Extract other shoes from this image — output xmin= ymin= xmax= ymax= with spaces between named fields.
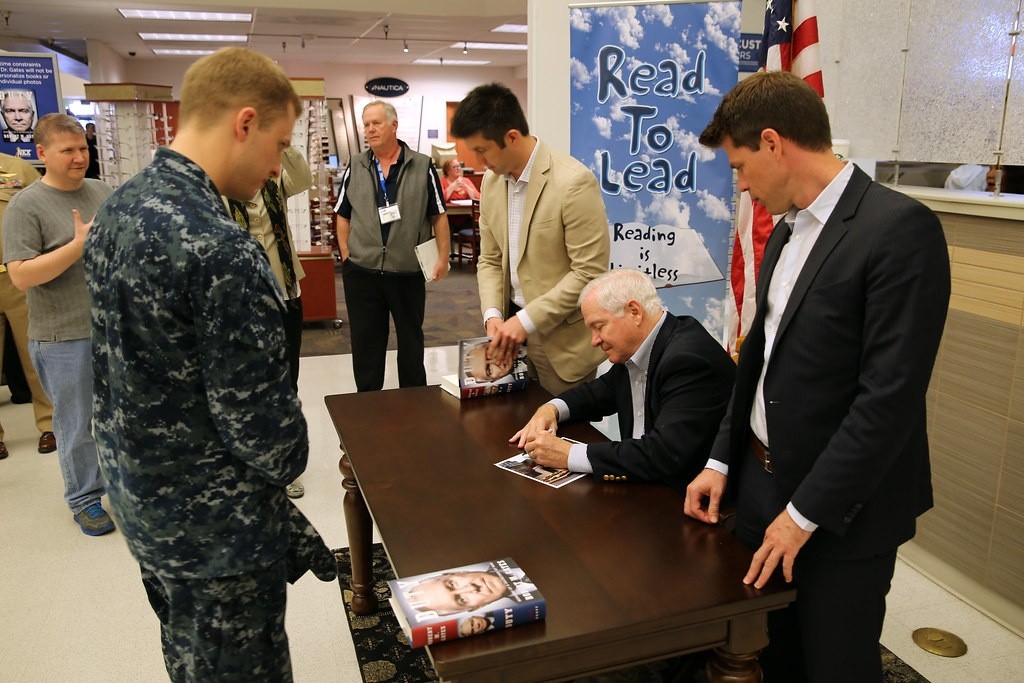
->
xmin=286 ymin=480 xmax=305 ymax=499
xmin=10 ymin=395 xmax=33 ymax=404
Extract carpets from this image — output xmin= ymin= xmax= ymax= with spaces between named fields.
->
xmin=330 ymin=538 xmax=934 ymax=683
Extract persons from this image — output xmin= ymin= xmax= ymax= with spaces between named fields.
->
xmin=684 ymin=71 xmax=950 ymax=683
xmin=408 ymin=559 xmax=542 ymax=616
xmin=220 ymin=139 xmax=313 ymax=499
xmin=450 ymin=79 xmax=611 ymax=396
xmin=333 ymin=98 xmax=451 ymax=393
xmin=459 ymin=616 xmax=490 ymax=637
xmin=83 ymin=46 xmax=310 ymax=683
xmin=85 ymin=123 xmax=101 ymax=180
xmin=0 ymin=153 xmax=57 ymax=460
xmin=2 ymin=113 xmax=115 ymax=536
xmin=985 ymin=165 xmax=1024 ymax=194
xmin=0 ymin=92 xmax=36 ymax=132
xmin=465 ymin=340 xmax=527 ymax=384
xmin=508 ymin=268 xmax=737 ymax=493
xmin=440 ymin=157 xmax=480 ymax=202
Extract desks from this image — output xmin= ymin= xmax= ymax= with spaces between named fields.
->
xmin=321 ymin=376 xmax=802 ymax=682
xmin=443 ymin=200 xmax=483 ymax=264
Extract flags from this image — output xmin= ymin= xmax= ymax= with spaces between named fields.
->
xmin=731 ymin=0 xmax=826 ymax=351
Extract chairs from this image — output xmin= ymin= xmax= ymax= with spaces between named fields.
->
xmin=450 ymin=200 xmax=480 ymax=272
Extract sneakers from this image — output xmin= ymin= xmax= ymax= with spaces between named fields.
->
xmin=73 ymin=503 xmax=116 ymax=536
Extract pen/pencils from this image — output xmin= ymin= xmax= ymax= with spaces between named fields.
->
xmin=522 ymin=429 xmax=553 ymax=456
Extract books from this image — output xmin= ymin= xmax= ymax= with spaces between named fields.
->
xmin=386 ymin=557 xmax=547 ymax=650
xmin=439 ymin=337 xmax=527 ymax=400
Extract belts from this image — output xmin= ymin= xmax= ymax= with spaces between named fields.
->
xmin=752 ymin=441 xmax=773 ymax=474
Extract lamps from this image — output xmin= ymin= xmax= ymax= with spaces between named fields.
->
xmin=402 ymin=37 xmax=409 ymax=53
xmin=463 ymin=41 xmax=468 ymax=55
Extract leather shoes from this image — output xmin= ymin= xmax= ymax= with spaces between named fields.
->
xmin=0 ymin=442 xmax=8 ymax=460
xmin=38 ymin=432 xmax=56 ymax=453
